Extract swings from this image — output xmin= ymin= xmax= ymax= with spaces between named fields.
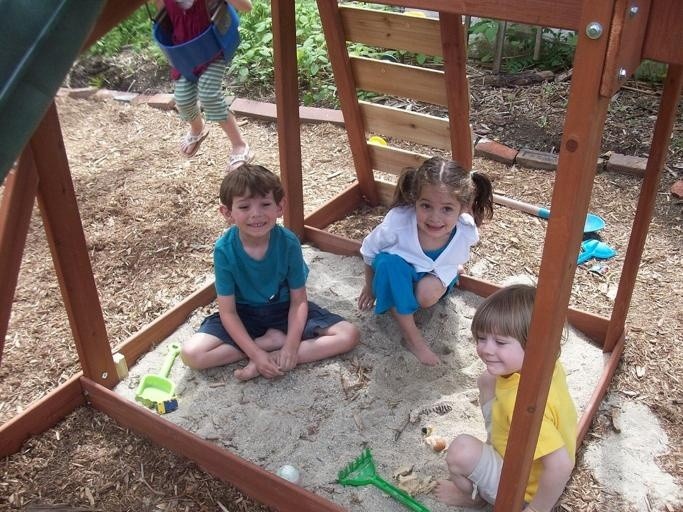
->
xmin=144 ymin=0 xmax=238 ymax=80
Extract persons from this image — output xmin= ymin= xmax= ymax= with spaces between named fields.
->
xmin=178 ymin=156 xmax=360 ymax=382
xmin=431 ymin=284 xmax=580 ymax=512
xmin=151 ymin=0 xmax=255 ymax=175
xmin=358 ymin=155 xmax=495 ymax=367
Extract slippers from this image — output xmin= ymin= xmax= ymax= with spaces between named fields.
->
xmin=181 ymin=119 xmax=210 ymax=158
xmin=226 ymin=142 xmax=255 ymax=173
